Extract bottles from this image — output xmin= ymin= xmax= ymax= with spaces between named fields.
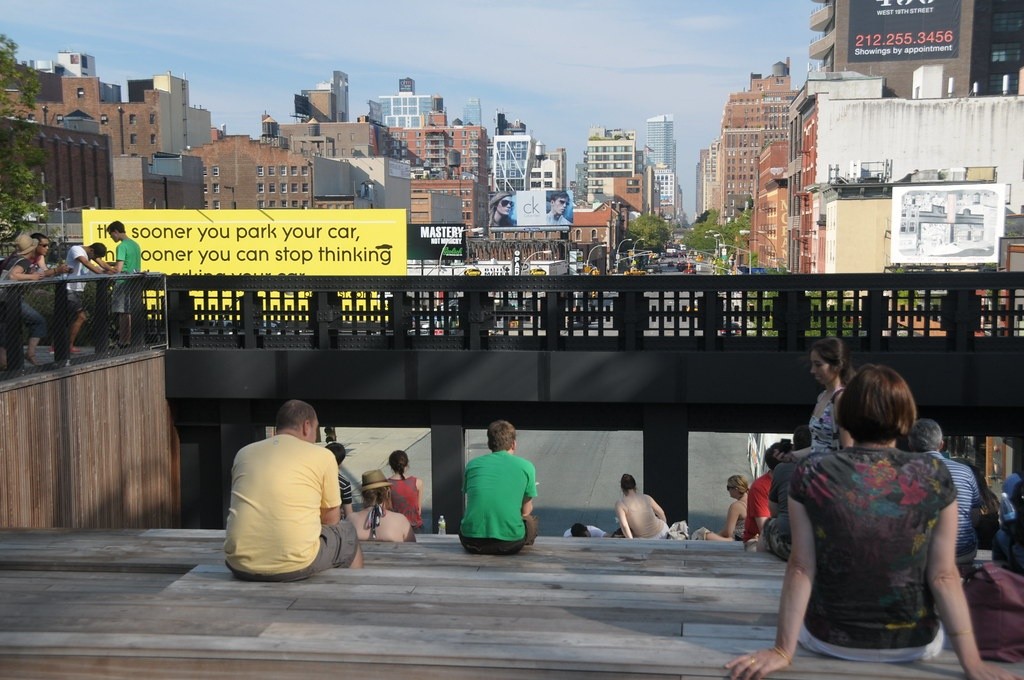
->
xmin=60 ymin=259 xmax=67 ymax=278
xmin=36 ymin=265 xmax=45 ymax=273
xmin=438 ymin=515 xmax=446 ymax=536
xmin=1001 ymin=493 xmax=1016 ymax=521
xmin=994 ymin=440 xmax=999 ymax=451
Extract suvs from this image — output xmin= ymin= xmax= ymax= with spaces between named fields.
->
xmin=647 ymin=265 xmax=661 ymax=273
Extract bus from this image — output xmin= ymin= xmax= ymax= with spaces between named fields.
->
xmin=680 ymin=245 xmax=686 ymax=251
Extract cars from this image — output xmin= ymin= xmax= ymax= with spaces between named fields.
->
xmin=721 ymin=323 xmax=740 ymax=336
xmin=624 ymin=267 xmax=646 ymax=275
xmin=668 ymin=262 xmax=674 ymax=266
xmin=572 ymin=315 xmax=592 ymax=329
xmin=683 ymin=262 xmax=696 ymax=274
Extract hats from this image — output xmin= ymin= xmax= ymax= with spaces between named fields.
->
xmin=15 ymin=233 xmax=39 ymax=254
xmin=358 ymin=469 xmax=391 ymax=490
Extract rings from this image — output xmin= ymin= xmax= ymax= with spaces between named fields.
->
xmin=750 ymin=657 xmax=756 ymax=665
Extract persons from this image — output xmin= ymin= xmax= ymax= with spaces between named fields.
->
xmin=725 ymin=364 xmax=1024 ymax=680
xmin=616 ymin=475 xmax=670 ymax=539
xmin=324 ymin=442 xmax=352 ymax=523
xmin=706 ymin=476 xmax=751 ymax=540
xmin=487 ymin=191 xmax=517 ymax=227
xmin=384 ymin=450 xmax=425 ymax=534
xmin=51 ymin=243 xmax=119 ymax=356
xmin=224 ymin=399 xmax=363 ymax=582
xmin=742 ymin=338 xmax=1023 ymax=576
xmin=562 ymin=522 xmax=610 ymax=538
xmin=460 ymin=421 xmax=538 ymax=555
xmin=546 ymin=190 xmax=573 ymax=225
xmin=345 ymin=470 xmax=416 ymax=543
xmin=0 ymin=233 xmax=70 ymax=371
xmin=106 ymin=221 xmax=141 ymax=350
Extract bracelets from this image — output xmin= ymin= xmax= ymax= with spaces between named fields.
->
xmin=53 ymin=269 xmax=57 ymax=273
xmin=773 ymin=647 xmax=792 ymax=665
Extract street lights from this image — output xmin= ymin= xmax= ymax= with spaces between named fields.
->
xmin=705 ymin=230 xmax=725 ymax=274
xmin=617 ymin=239 xmax=633 ymax=274
xmin=521 ymin=250 xmax=551 ymax=274
xmin=740 ymin=230 xmax=779 ymax=272
xmin=633 ymin=238 xmax=644 ymax=267
xmin=719 ymin=244 xmax=752 ymax=274
xmin=587 ymin=244 xmax=607 ymax=275
xmin=439 ymin=227 xmax=484 ymax=276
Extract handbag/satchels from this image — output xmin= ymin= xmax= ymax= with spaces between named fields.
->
xmin=0 ymin=258 xmax=29 ymax=302
xmin=957 ymin=560 xmax=1024 ymax=662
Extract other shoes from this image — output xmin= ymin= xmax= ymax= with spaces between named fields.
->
xmin=69 ymin=345 xmax=82 ymax=354
xmin=50 ymin=347 xmax=54 ymax=353
xmin=25 ymin=352 xmax=40 ymax=366
xmin=110 ymin=343 xmax=129 ymax=349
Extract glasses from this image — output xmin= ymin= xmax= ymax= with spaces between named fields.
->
xmin=557 ymin=199 xmax=571 ymax=206
xmin=501 ymin=199 xmax=515 ymax=209
xmin=726 ymin=486 xmax=736 ymax=490
xmin=38 ymin=243 xmax=50 ymax=248
